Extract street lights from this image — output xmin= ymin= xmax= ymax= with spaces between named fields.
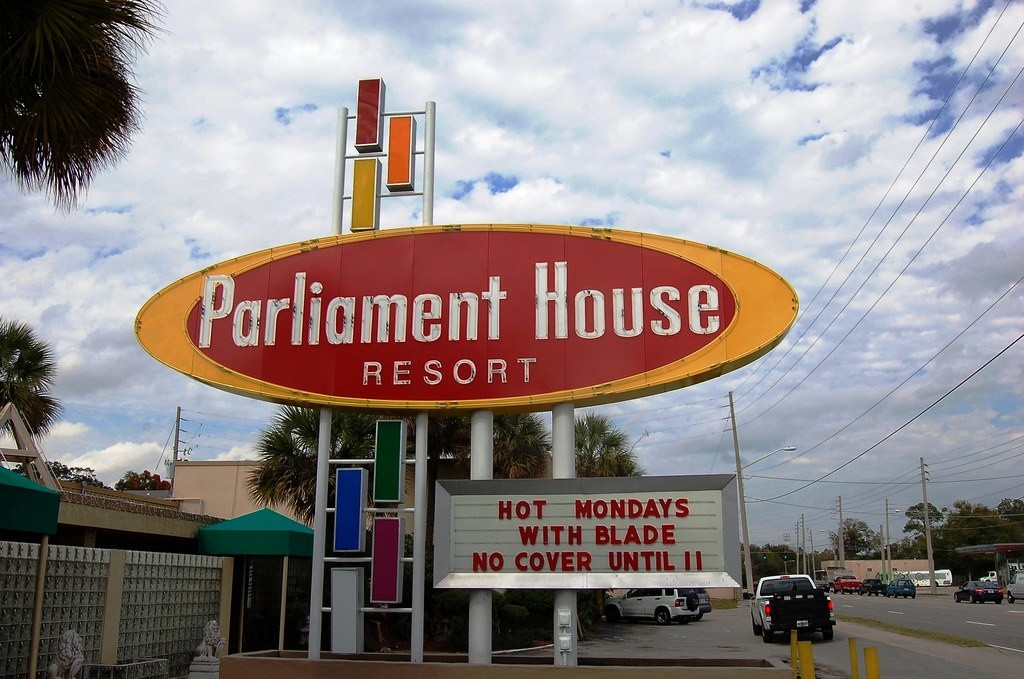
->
xmin=734 ymin=446 xmax=797 ymax=598
xmin=894 ymin=509 xmax=937 ymax=594
xmin=820 ymin=530 xmax=845 ymax=567
xmin=783 ymin=560 xmax=795 ymax=574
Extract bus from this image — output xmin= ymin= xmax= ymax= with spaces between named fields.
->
xmin=875 ymin=569 xmax=952 ymax=587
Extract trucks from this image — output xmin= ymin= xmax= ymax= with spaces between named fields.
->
xmin=1006 ymin=573 xmax=1024 ymax=604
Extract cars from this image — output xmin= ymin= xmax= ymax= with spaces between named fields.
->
xmin=886 ymin=579 xmax=916 ymax=598
xmin=815 ymin=580 xmax=830 ymax=593
xmin=954 ymin=581 xmax=1004 ymax=604
xmin=978 ymin=571 xmax=998 ymax=582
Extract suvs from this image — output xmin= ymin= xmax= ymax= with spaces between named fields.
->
xmin=603 ymin=588 xmax=712 ymax=626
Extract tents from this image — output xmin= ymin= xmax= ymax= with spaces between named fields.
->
xmin=197 ymin=508 xmax=313 ymax=653
xmin=0 ymin=465 xmax=61 ymax=679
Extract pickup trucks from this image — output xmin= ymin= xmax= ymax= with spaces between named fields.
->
xmin=858 ymin=579 xmax=888 ymax=596
xmin=833 ymin=575 xmax=863 ymax=594
xmin=750 ymin=574 xmax=836 ymax=643
